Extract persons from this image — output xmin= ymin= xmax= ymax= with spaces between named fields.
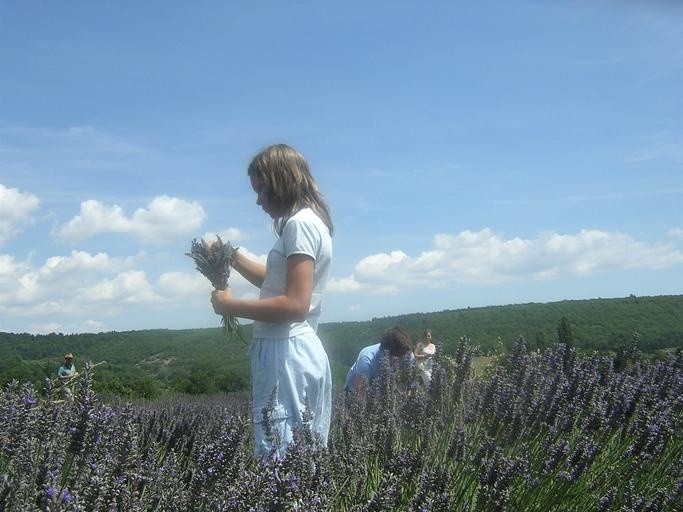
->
xmin=344 ymin=326 xmax=417 ymax=399
xmin=412 ymin=328 xmax=437 ymax=389
xmin=199 ymin=143 xmax=335 ymax=469
xmin=56 ymin=352 xmax=79 ymax=403
